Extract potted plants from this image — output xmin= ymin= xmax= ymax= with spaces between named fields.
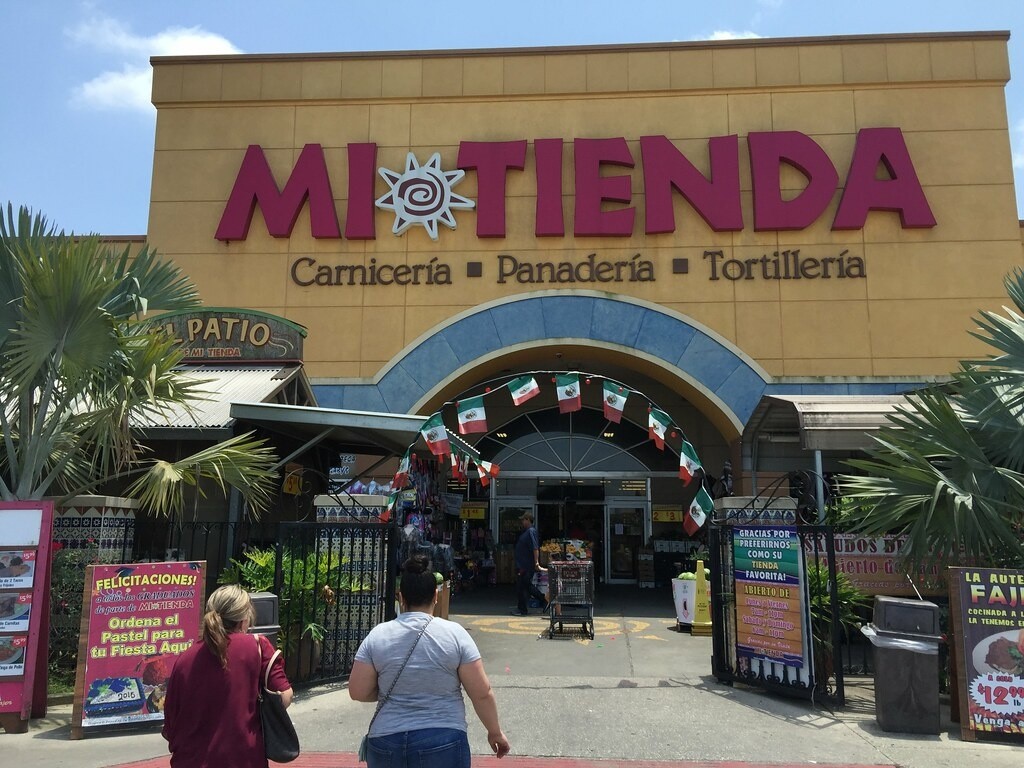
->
xmin=214 ymin=543 xmax=375 ymax=682
xmin=806 ymin=556 xmax=875 ymax=693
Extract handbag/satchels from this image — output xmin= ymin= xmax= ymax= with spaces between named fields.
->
xmin=514 ymin=568 xmax=526 ymax=577
xmin=252 ymin=632 xmax=299 ymax=764
xmin=356 ymin=735 xmax=367 ymax=761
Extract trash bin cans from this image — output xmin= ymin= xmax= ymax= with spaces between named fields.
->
xmin=248 ymin=591 xmax=283 ymax=651
xmin=869 ymin=593 xmax=945 ymax=736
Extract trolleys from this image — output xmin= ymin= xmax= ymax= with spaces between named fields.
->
xmin=546 ymin=560 xmax=596 ymax=639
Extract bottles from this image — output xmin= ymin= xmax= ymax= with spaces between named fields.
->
xmin=366 ymin=478 xmax=378 ymax=495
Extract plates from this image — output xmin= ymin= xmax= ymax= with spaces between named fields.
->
xmin=0 ymin=647 xmax=23 ymax=663
xmin=972 ymin=630 xmax=1021 ymax=675
xmin=0 ymin=594 xmax=28 ymax=620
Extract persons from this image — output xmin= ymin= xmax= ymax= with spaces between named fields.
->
xmin=590 ymin=522 xmax=605 ymax=582
xmin=538 ymin=553 xmax=563 ymax=616
xmin=163 ymin=584 xmax=293 ymax=768
xmin=512 ymin=511 xmax=550 ymax=617
xmin=559 ymin=519 xmax=588 ymax=540
xmin=349 ymin=554 xmax=510 ymax=768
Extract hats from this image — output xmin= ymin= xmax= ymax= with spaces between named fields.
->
xmin=518 ymin=512 xmax=535 ymax=520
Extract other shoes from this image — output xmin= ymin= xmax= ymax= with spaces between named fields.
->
xmin=543 ymin=601 xmax=550 ymax=613
xmin=512 ymin=611 xmax=528 ymax=617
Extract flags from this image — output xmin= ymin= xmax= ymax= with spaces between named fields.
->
xmin=648 ymin=404 xmax=673 ymax=451
xmin=439 ymin=444 xmax=500 ymax=487
xmin=603 ymin=380 xmax=630 ymax=424
xmin=683 ymin=488 xmax=713 ymax=537
xmin=679 ymin=440 xmax=706 ymax=487
xmin=420 ymin=413 xmax=451 ymax=455
xmin=377 ymin=447 xmax=410 ymax=525
xmin=555 ymin=373 xmax=582 ymax=414
xmin=507 ymin=373 xmax=540 ymax=407
xmin=455 ymin=397 xmax=488 ymax=435
